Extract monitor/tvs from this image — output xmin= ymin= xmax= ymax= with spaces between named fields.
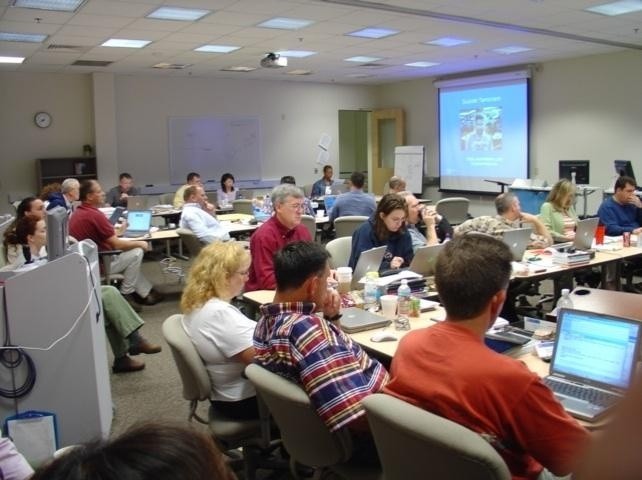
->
xmin=47 ymin=206 xmax=69 ymax=262
xmin=559 ymin=160 xmax=589 ymax=186
xmin=614 ymin=160 xmax=636 ymax=185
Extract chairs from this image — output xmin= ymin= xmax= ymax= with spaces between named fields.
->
xmin=0 ymin=179 xmax=642 ymax=322
xmin=243 ymin=355 xmax=361 ymax=480
xmin=360 ymin=391 xmax=515 ymax=480
xmin=159 ymin=307 xmax=316 ymax=480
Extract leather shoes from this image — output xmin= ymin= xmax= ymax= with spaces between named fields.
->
xmin=113 ymin=359 xmax=145 ymax=372
xmin=129 ymin=340 xmax=161 ymax=355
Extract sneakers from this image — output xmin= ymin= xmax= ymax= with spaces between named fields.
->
xmin=123 ymin=293 xmax=142 ymax=313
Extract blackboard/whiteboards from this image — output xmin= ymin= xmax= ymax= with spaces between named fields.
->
xmin=168 ymin=116 xmax=265 ymax=189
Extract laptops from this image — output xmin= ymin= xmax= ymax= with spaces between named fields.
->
xmin=327 ymin=245 xmax=388 ymax=291
xmin=314 ymin=307 xmax=392 ymax=334
xmin=549 ymin=216 xmax=600 ymax=250
xmin=324 ymin=195 xmax=338 ymax=215
xmin=502 ymin=228 xmax=533 ymax=261
xmin=127 ymin=197 xmax=148 ymax=210
xmin=540 ymin=308 xmax=642 ymax=422
xmin=123 ymin=210 xmax=152 ymax=237
xmin=332 ymin=185 xmax=348 ymax=195
xmin=109 ymin=208 xmax=126 ymax=226
xmin=397 ymin=243 xmax=445 ymax=276
xmin=235 ymin=191 xmax=253 ymax=200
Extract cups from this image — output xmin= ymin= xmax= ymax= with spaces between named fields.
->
xmin=336 ymin=266 xmax=353 ymax=294
xmin=595 ymin=224 xmax=606 ymax=245
xmin=317 ymin=209 xmax=325 ymax=219
xmin=380 ymin=293 xmax=398 ymax=317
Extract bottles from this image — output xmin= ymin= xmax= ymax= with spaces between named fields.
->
xmin=263 ymin=194 xmax=273 ymax=219
xmin=397 ymin=279 xmax=412 ymax=319
xmin=251 ymin=196 xmax=265 ymax=220
xmin=554 ymin=286 xmax=574 ymax=319
xmin=362 ymin=272 xmax=379 ymax=307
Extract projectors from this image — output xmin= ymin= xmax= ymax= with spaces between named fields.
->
xmin=261 ymin=56 xmax=287 ymax=68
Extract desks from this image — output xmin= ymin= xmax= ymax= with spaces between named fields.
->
xmin=235 ymin=284 xmax=641 ymax=437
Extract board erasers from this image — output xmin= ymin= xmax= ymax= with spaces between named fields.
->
xmin=145 ymin=184 xmax=153 ymax=188
xmin=207 ymin=179 xmax=214 ymax=183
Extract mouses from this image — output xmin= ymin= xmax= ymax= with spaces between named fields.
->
xmin=371 ymin=333 xmax=397 ymax=342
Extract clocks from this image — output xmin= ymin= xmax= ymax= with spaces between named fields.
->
xmin=33 ymin=111 xmax=53 ymax=129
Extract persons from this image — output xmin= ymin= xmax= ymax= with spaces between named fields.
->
xmin=459 ymin=112 xmax=503 ymax=151
xmin=29 ymin=416 xmax=239 ymax=480
xmin=180 ymin=238 xmax=265 ymax=422
xmin=382 ymin=232 xmax=590 ymax=480
xmin=5 ymin=164 xmax=641 ymax=377
xmin=252 ymin=239 xmax=390 ymax=480
